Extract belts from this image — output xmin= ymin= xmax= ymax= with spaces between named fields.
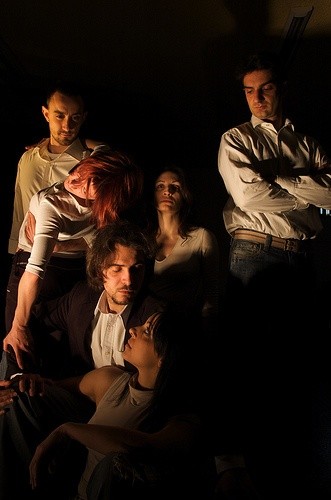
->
xmin=234 ymin=229 xmax=298 ymax=251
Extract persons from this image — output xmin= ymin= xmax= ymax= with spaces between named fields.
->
xmin=0 ymin=57 xmax=331 ymax=500
